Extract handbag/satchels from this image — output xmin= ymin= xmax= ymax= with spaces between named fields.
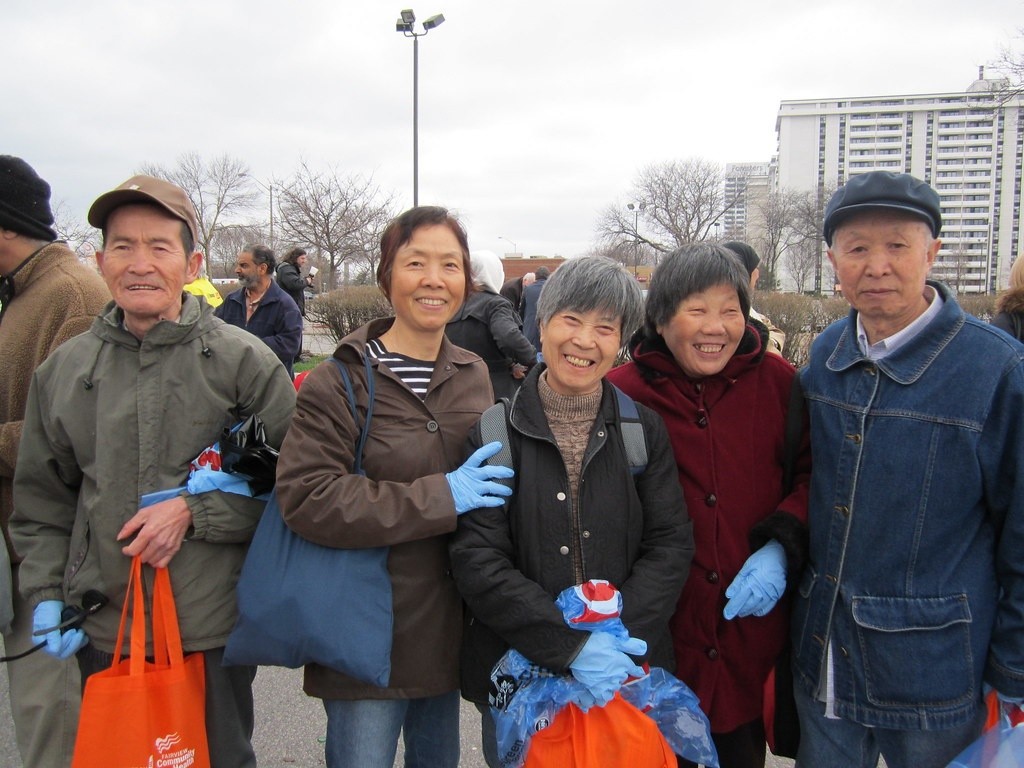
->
xmin=764 ymin=645 xmax=800 ymax=760
xmin=70 ymin=554 xmax=212 ymax=768
xmin=221 ymin=356 xmax=393 ymax=692
xmin=944 ymin=690 xmax=1024 ymax=768
xmin=519 ymin=663 xmax=680 ymax=768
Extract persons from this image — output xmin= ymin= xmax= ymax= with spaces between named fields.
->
xmin=213 ymin=242 xmax=312 ymax=381
xmin=796 ymin=172 xmax=1024 ymax=768
xmin=604 ymin=240 xmax=810 ymax=768
xmin=7 ymin=175 xmax=314 ymax=768
xmin=0 ymin=156 xmax=114 ymax=768
xmin=276 ymin=206 xmax=692 ymax=768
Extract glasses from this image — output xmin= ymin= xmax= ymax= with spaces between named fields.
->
xmin=0 ymin=588 xmax=110 ymax=663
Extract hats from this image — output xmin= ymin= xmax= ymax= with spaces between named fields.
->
xmin=823 ymin=170 xmax=942 ymax=248
xmin=88 ymin=175 xmax=200 ymax=249
xmin=1 ymin=155 xmax=58 ymax=242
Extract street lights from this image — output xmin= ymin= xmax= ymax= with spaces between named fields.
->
xmin=396 ymin=9 xmax=445 ymax=208
xmin=498 ymin=236 xmax=516 ymax=254
xmin=714 ymin=223 xmax=720 ymax=241
xmin=627 ymin=203 xmax=646 ymax=279
xmin=238 ymin=173 xmax=274 ymax=251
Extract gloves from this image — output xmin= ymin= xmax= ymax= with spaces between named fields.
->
xmin=570 ymin=631 xmax=648 ymax=703
xmin=443 ymin=441 xmax=516 ymax=515
xmin=724 ymin=540 xmax=788 ymax=618
xmin=31 ymin=600 xmax=90 ymax=659
